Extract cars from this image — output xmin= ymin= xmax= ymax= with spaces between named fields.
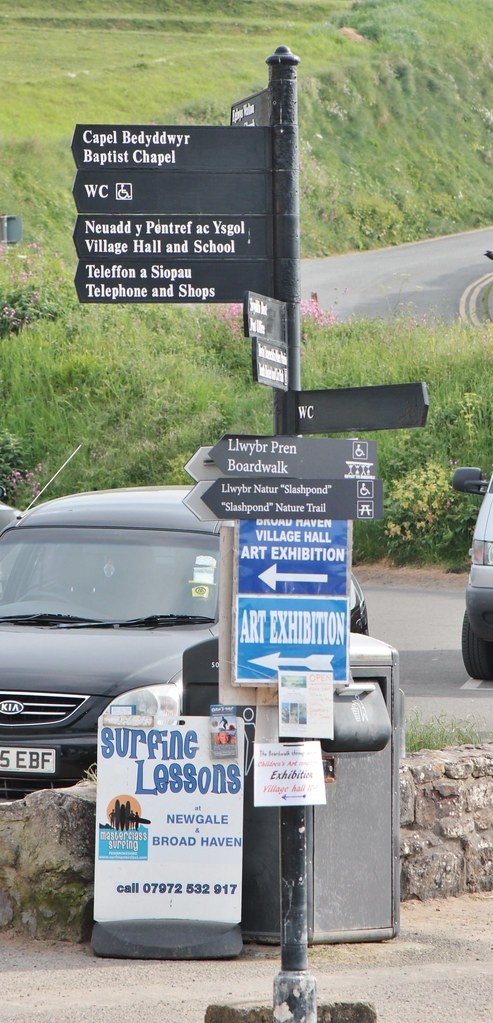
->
xmin=0 ymin=485 xmax=370 ymax=802
xmin=451 ymin=466 xmax=493 ymax=682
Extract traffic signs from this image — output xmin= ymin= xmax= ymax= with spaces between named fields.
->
xmin=180 ymin=432 xmax=379 ymax=478
xmin=179 ymin=478 xmax=384 ymax=521
xmin=69 ymin=124 xmax=277 ymax=302
xmin=230 ymin=594 xmax=352 ymax=684
xmin=236 ymin=520 xmax=354 ymax=598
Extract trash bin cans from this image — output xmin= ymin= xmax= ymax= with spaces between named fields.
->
xmin=241 ymin=632 xmax=405 ymax=948
